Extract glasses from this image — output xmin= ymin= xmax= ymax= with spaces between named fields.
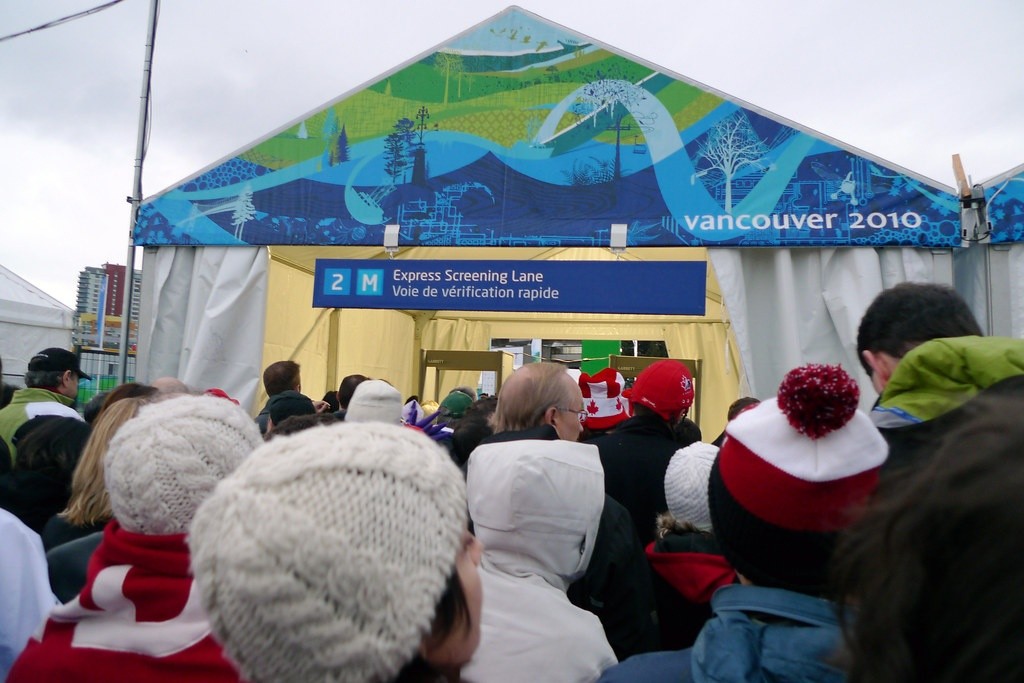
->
xmin=541 ymin=407 xmax=589 ymax=422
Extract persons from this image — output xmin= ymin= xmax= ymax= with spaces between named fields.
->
xmin=0 ymin=283 xmax=1024 ymax=683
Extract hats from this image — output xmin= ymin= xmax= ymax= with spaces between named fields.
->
xmin=27 ymin=347 xmax=93 ymax=382
xmin=203 ymin=388 xmax=240 ymax=406
xmin=707 ymin=363 xmax=889 ymax=593
xmin=439 ymin=392 xmax=475 ymax=419
xmin=267 ymin=391 xmax=317 ymax=427
xmin=577 ymin=367 xmax=631 ymax=427
xmin=344 ymin=379 xmax=402 ymax=425
xmin=186 ymin=419 xmax=465 ymax=683
xmin=104 ymin=393 xmax=264 ymax=536
xmin=663 ymin=442 xmax=719 ymax=533
xmin=622 ymin=358 xmax=694 ymax=427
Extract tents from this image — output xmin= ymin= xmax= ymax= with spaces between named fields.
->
xmin=0 ymin=6 xmax=1024 ymax=404
